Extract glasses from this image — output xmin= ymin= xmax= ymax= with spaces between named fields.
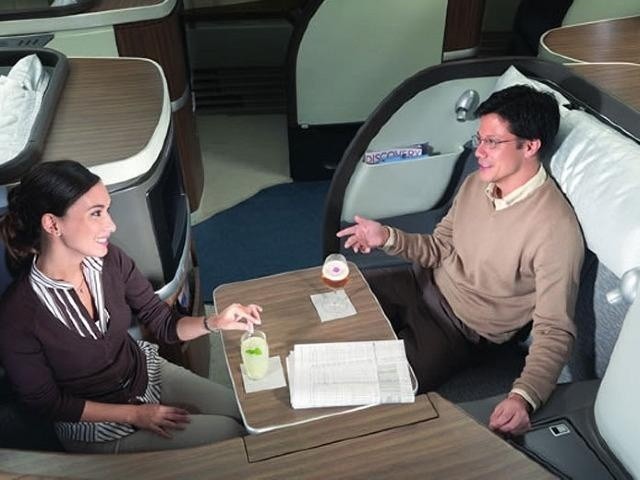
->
xmin=471 ymin=134 xmax=520 ymax=149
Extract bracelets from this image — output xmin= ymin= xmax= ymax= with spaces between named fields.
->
xmin=203 ymin=317 xmax=215 ymax=333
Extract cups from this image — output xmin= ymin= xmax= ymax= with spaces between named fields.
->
xmin=241 ymin=330 xmax=272 ymax=379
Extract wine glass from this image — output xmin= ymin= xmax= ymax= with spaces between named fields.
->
xmin=320 ymin=254 xmax=351 ymax=311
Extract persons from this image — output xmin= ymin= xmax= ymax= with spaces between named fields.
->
xmin=1 ymin=159 xmax=264 ymax=455
xmin=336 ymin=83 xmax=585 ymax=437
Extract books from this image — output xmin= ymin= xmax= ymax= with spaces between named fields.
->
xmin=365 ymin=144 xmax=422 ymax=163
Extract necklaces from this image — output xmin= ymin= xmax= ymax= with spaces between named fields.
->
xmin=75 ymin=278 xmax=86 ymax=294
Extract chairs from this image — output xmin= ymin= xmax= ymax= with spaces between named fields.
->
xmin=324 ymin=57 xmax=639 ymax=404
